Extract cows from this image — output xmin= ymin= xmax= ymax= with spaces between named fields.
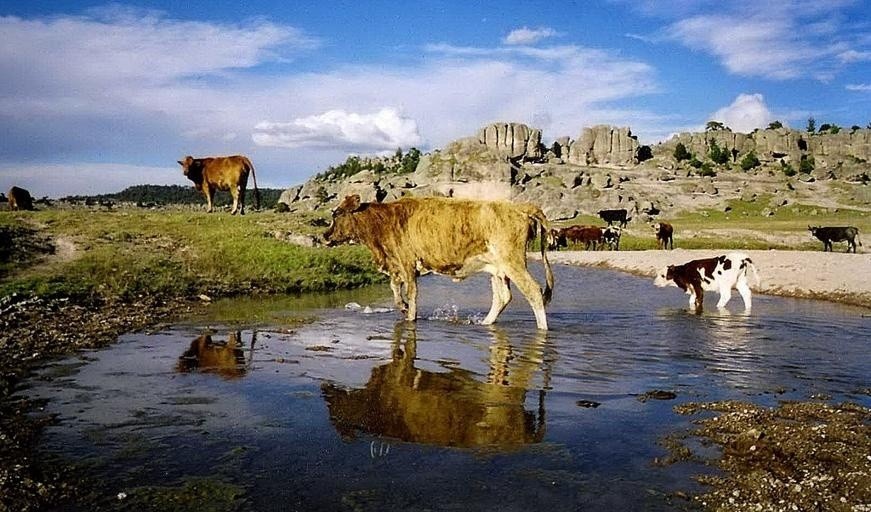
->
xmin=597 ymin=209 xmax=632 ymax=228
xmin=169 ymin=325 xmax=257 ymax=382
xmin=545 ymin=225 xmax=622 ymax=251
xmin=177 ymin=156 xmax=261 ymax=215
xmin=319 ymin=317 xmax=552 ymax=454
xmin=807 ymin=224 xmax=862 ymax=253
xmin=8 ymin=185 xmax=33 ymax=212
xmin=320 ymin=193 xmax=555 ymax=332
xmin=653 ymin=253 xmax=761 ymax=311
xmin=651 ymin=222 xmax=673 ymax=250
